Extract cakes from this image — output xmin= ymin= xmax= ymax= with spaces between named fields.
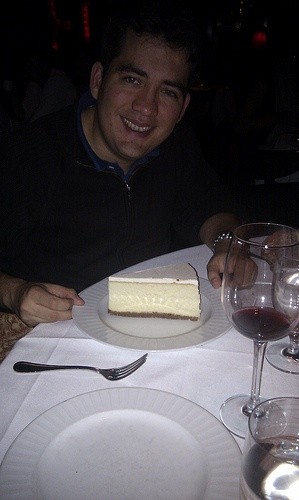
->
xmin=107 ymin=263 xmax=201 ymax=322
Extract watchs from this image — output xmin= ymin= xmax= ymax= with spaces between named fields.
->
xmin=212 ymin=231 xmax=250 ymax=253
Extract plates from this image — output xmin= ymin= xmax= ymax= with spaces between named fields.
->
xmin=72 ymin=277 xmax=234 ymax=352
xmin=0 ymin=386 xmax=245 ymax=500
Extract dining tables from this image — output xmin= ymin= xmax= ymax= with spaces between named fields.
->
xmin=0 ymin=234 xmax=299 ymax=500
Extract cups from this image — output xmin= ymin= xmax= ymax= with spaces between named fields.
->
xmin=240 ymin=397 xmax=299 ymax=500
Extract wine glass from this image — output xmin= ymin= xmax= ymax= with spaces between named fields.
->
xmin=220 ymin=223 xmax=299 ymax=439
xmin=265 ymin=252 xmax=299 ymax=374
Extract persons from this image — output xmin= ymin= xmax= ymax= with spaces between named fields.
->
xmin=0 ymin=12 xmax=258 ymax=327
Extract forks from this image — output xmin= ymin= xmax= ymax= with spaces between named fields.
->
xmin=13 ymin=353 xmax=148 ymax=381
